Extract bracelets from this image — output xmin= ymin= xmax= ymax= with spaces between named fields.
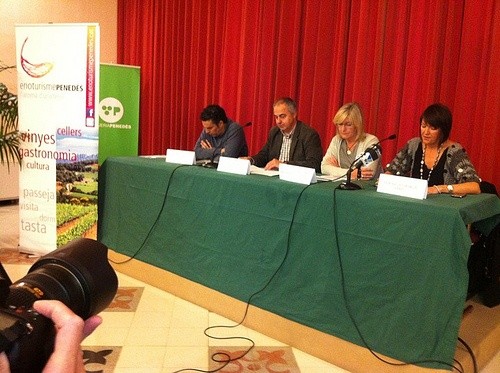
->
xmin=434 ymin=184 xmax=442 ymax=193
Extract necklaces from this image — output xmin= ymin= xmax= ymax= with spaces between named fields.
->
xmin=420 ymin=142 xmax=443 ymax=181
xmin=346 ymin=140 xmax=358 ymax=155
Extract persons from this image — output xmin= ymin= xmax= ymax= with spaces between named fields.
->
xmin=383 ymin=103 xmax=481 ymax=195
xmin=0 ymin=300 xmax=103 ymax=373
xmin=321 ymin=103 xmax=382 ymax=181
xmin=194 ymin=104 xmax=248 ymax=160
xmin=239 ymin=97 xmax=323 ymax=174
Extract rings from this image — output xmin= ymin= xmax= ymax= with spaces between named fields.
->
xmin=362 ymin=175 xmax=364 ymax=178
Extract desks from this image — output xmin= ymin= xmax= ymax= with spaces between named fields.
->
xmin=96 ymin=154 xmax=500 ymax=370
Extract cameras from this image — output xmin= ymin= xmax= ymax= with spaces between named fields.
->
xmin=0 ymin=236 xmax=119 ymax=373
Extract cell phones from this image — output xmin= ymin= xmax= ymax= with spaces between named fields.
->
xmin=451 ymin=193 xmax=468 ymax=198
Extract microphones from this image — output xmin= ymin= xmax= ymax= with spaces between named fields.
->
xmin=350 ymin=144 xmax=383 ymax=172
xmin=336 ymin=134 xmax=396 ymax=190
xmin=202 ymin=121 xmax=253 ymax=168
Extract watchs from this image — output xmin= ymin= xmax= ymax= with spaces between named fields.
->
xmin=447 ymin=183 xmax=453 ymax=193
